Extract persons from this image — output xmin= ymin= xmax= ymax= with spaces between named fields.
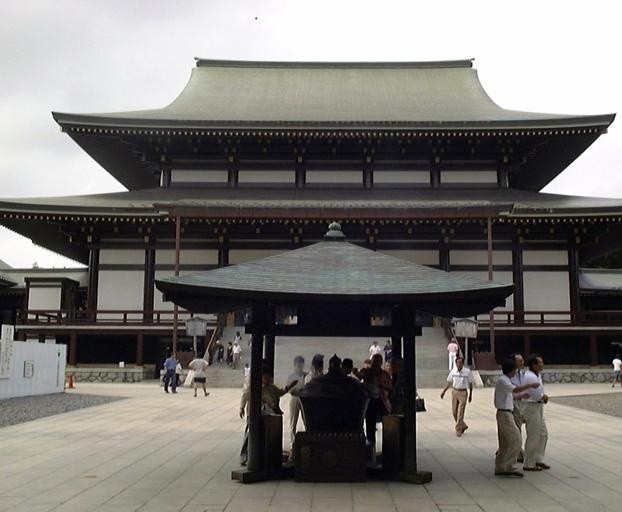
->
xmin=164 ymin=330 xmax=393 ymax=467
xmin=493 ymin=358 xmax=541 ymax=477
xmin=519 ymin=355 xmax=550 ymax=471
xmin=446 ymin=337 xmax=459 ymax=370
xmin=612 ymin=354 xmax=622 ymax=388
xmin=440 ymin=356 xmax=474 ymax=437
xmin=494 ymin=354 xmax=526 ymax=462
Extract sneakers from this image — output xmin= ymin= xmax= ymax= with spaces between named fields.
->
xmin=495 ymin=471 xmax=524 ymax=479
xmin=457 ymin=426 xmax=469 ymax=437
xmin=523 ymin=462 xmax=550 ymax=471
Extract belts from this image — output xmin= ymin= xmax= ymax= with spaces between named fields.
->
xmin=497 ymin=408 xmax=514 ymax=415
xmin=452 ymin=387 xmax=467 ymax=392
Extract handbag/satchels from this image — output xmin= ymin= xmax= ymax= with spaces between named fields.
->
xmin=415 ymin=394 xmax=426 ymax=413
